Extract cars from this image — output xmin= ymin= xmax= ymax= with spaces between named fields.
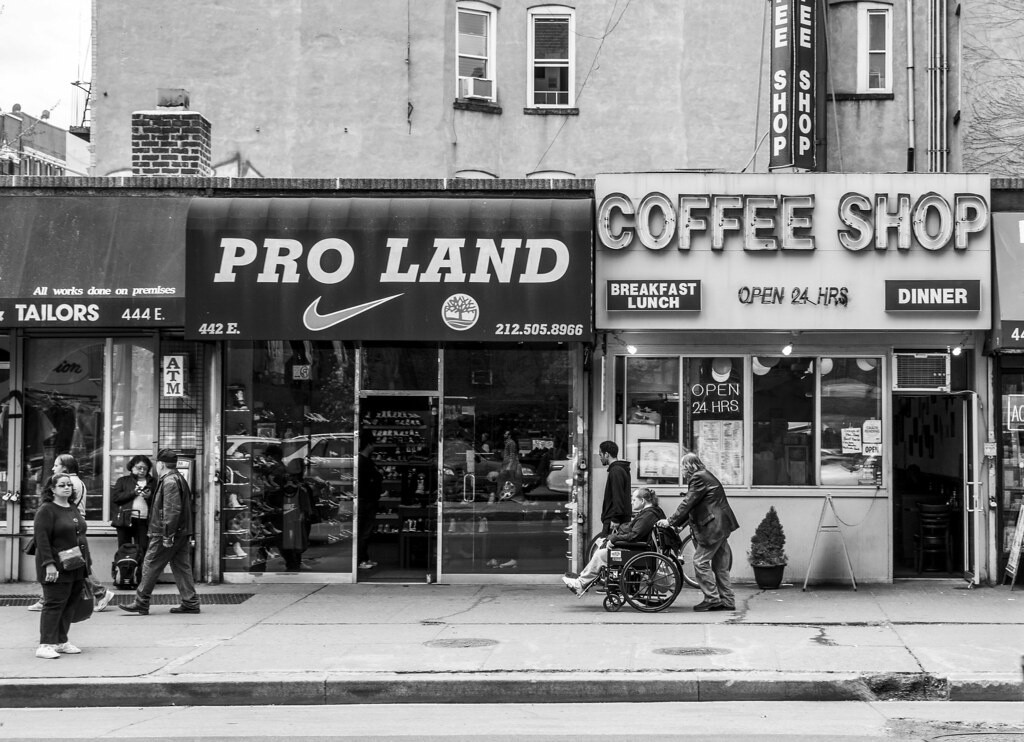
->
xmin=29 ymin=432 xmax=353 ymax=546
xmin=442 ymin=441 xmax=540 ymax=502
xmin=545 ymin=443 xmax=688 ymax=493
xmin=443 ymin=461 xmax=465 ymax=495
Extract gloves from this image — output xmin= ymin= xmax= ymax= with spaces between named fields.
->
xmin=267 ymin=340 xmax=285 ymax=374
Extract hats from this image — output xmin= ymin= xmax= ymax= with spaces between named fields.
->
xmin=151 ymin=448 xmax=177 ymax=464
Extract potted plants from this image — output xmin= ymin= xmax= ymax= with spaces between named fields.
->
xmin=747 ymin=505 xmax=788 ymax=589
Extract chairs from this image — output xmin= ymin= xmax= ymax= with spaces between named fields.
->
xmin=911 ymin=501 xmax=953 ymax=573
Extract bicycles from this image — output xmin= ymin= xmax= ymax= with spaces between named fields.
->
xmin=586 ymin=492 xmax=733 ymax=589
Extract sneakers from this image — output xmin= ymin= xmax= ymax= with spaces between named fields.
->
xmin=55 ymin=641 xmax=81 ymax=654
xmin=119 ymin=600 xmax=150 ymax=614
xmin=94 ymin=590 xmax=114 ymax=612
xmin=36 ymin=644 xmax=60 ymax=658
xmin=27 ymin=602 xmax=44 ymax=610
xmin=562 ymin=577 xmax=584 ymax=597
xmin=170 ymin=603 xmax=200 ymax=614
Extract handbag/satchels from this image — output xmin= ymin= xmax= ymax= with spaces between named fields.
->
xmin=57 ymin=545 xmax=86 ymax=570
xmin=111 ymin=510 xmax=133 ymax=529
xmin=70 ymin=581 xmax=93 ymax=623
xmin=23 ymin=538 xmax=37 ymax=556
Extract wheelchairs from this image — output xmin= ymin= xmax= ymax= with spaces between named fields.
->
xmin=599 ymin=522 xmax=685 ymax=613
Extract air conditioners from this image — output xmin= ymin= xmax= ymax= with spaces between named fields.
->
xmin=462 ymin=77 xmax=493 ymax=100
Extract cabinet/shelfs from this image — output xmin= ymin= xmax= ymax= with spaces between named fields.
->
xmin=304 ymin=457 xmax=438 ymax=537
xmin=221 ymin=408 xmax=279 ymax=569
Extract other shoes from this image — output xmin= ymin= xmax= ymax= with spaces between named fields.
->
xmin=708 ymin=603 xmax=735 ymax=611
xmin=595 ymin=588 xmax=609 ymax=594
xmin=223 ymin=385 xmax=518 ymax=570
xmin=693 ymin=599 xmax=723 ymax=611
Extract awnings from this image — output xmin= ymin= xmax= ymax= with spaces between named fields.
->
xmin=0 ymin=195 xmax=593 ymax=342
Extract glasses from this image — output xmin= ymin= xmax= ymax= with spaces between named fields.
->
xmin=54 ymin=483 xmax=74 ymax=489
xmin=134 ymin=465 xmax=149 ymax=472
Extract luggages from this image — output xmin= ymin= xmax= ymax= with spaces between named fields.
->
xmin=111 ymin=508 xmax=144 ymax=590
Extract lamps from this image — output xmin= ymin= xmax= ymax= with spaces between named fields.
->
xmin=613 ymin=333 xmax=637 ymax=354
xmin=952 ymin=337 xmax=969 ymax=355
xmin=711 ymin=357 xmax=731 ymax=382
xmin=752 ymin=356 xmax=781 ymax=375
xmin=782 ymin=341 xmax=794 ymax=355
xmin=855 ymin=358 xmax=878 ymax=371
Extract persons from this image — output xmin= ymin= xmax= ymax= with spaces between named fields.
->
xmin=492 ymin=431 xmax=525 ymax=504
xmin=358 ymin=430 xmax=382 ymax=569
xmin=655 ymin=453 xmax=740 ymax=611
xmin=111 ymin=454 xmax=157 ymax=554
xmin=28 ymin=454 xmax=114 ymax=611
xmin=33 ymin=473 xmax=92 ymax=658
xmin=119 ymin=448 xmax=201 ymax=615
xmin=561 ymin=488 xmax=666 ymax=593
xmin=598 ymin=441 xmax=631 ymax=593
